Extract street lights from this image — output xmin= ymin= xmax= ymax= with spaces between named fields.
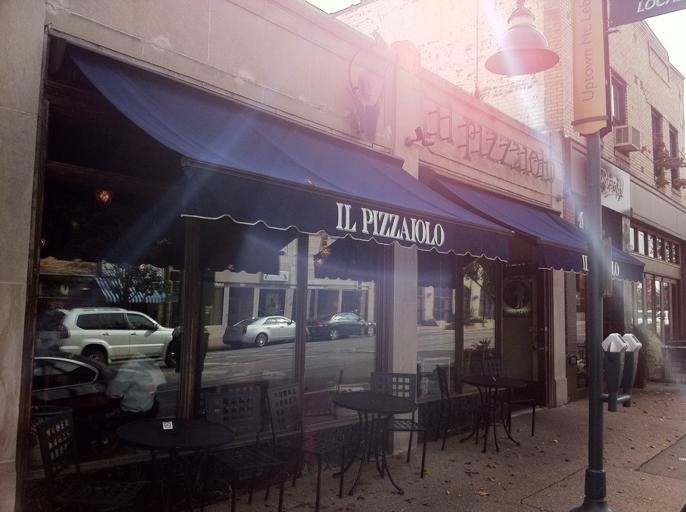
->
xmin=485 ymin=0 xmax=615 ymax=509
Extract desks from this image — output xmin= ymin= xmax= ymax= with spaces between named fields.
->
xmin=114 ymin=419 xmax=232 ymax=512
xmin=331 ymin=391 xmax=416 ymax=496
xmin=462 ymin=376 xmax=526 ymax=452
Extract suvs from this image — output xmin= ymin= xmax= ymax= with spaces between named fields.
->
xmin=37 ymin=304 xmax=181 ymax=369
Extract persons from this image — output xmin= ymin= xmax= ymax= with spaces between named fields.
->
xmin=85 ymin=334 xmax=168 ymax=447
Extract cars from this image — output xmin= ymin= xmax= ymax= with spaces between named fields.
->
xmin=30 ymin=354 xmax=155 ymax=413
xmin=310 ymin=310 xmax=379 ymax=340
xmin=227 ymin=315 xmax=310 ymax=345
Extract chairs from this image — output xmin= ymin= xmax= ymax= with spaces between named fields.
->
xmin=495 ymin=366 xmax=537 ymax=436
xmin=202 ymin=381 xmax=284 ymax=512
xmin=436 ymin=365 xmax=489 ymax=453
xmin=369 ymin=373 xmax=427 ymax=478
xmin=265 ymin=383 xmax=342 ymax=512
xmin=38 ymin=416 xmax=152 ymax=512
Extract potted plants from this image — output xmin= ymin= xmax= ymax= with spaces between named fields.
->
xmin=627 ymin=322 xmax=662 ymax=387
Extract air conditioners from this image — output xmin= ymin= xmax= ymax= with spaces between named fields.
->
xmin=613 ymin=124 xmax=641 ymax=152
